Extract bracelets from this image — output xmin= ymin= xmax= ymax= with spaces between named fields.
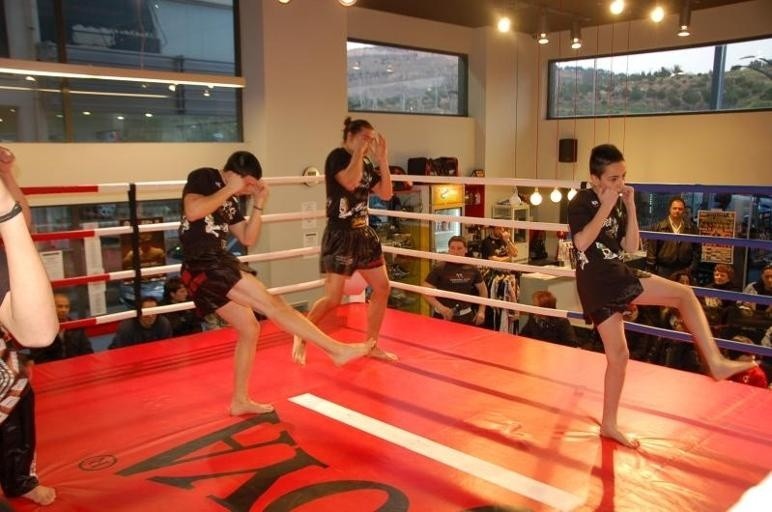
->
xmin=0 ymin=201 xmax=22 ymax=223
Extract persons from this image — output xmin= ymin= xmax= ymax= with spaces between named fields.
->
xmin=0 ymin=176 xmax=63 ymax=351
xmin=204 ymin=230 xmax=248 ymax=331
xmin=1 ymin=142 xmax=64 ymax=505
xmin=519 ymin=290 xmax=577 ymax=347
xmin=26 ymin=291 xmax=93 ymax=364
xmin=369 ymin=206 xmax=415 ymax=307
xmin=289 ymin=118 xmax=398 ymax=367
xmin=178 ymin=151 xmax=375 ymax=417
xmin=565 ymin=144 xmax=759 ymax=450
xmin=423 ymin=226 xmax=509 ymax=327
xmin=109 ymin=237 xmax=202 ymax=349
xmin=622 ymin=198 xmax=772 ymax=371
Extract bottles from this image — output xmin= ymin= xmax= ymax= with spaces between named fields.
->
xmin=451 ymin=304 xmax=460 ymax=312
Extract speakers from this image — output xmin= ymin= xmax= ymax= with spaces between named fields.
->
xmin=558 ymin=138 xmax=577 ymax=163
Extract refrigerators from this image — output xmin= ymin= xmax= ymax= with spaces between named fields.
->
xmin=389 ymin=184 xmax=467 ymax=313
